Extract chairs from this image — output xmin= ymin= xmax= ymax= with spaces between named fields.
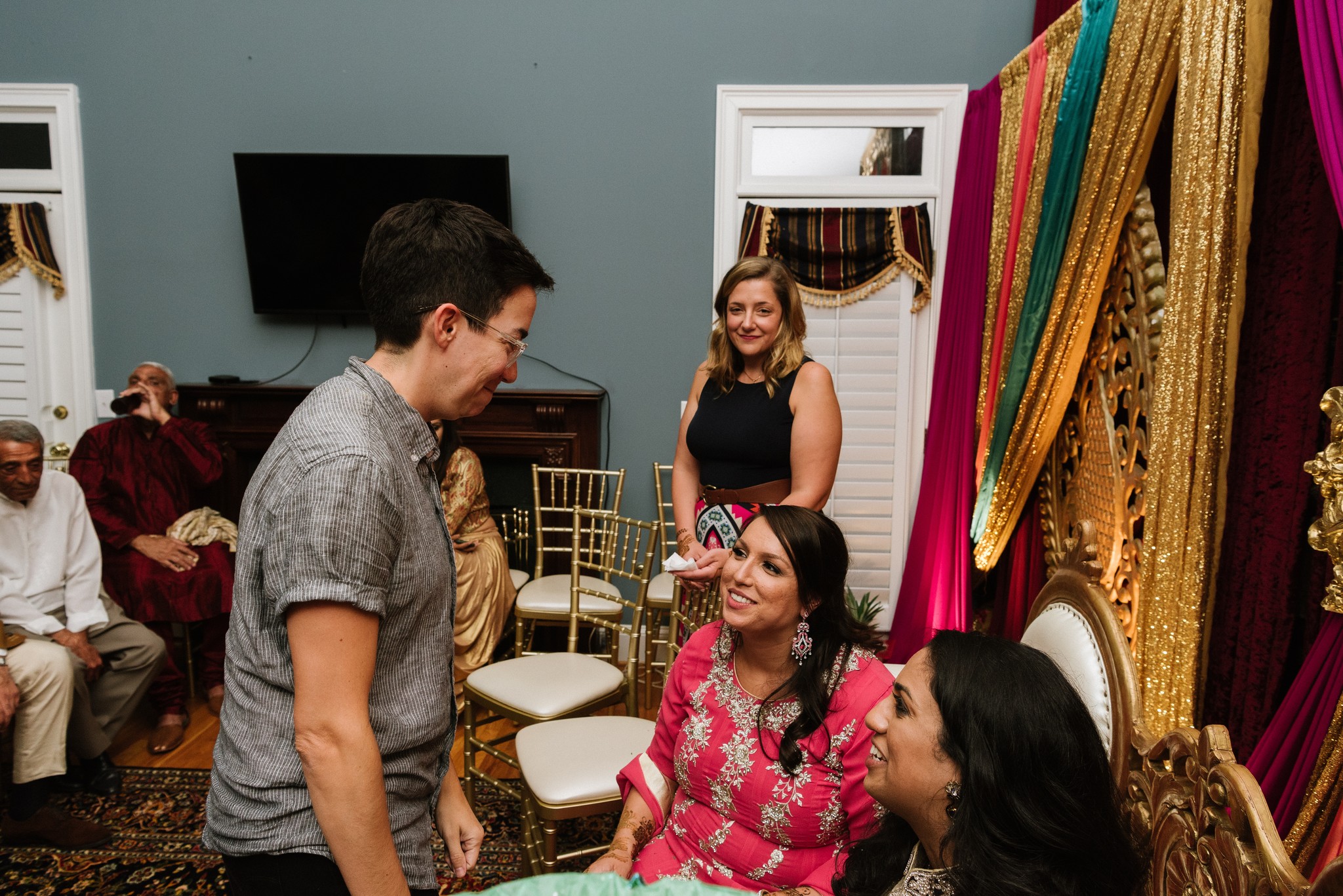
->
xmin=644 ymin=462 xmax=677 ymax=710
xmin=463 ymin=506 xmax=661 ymax=842
xmin=515 ymin=464 xmax=626 ymax=664
xmin=514 ymin=573 xmax=723 ymax=876
xmin=988 ymin=569 xmax=1343 ymax=895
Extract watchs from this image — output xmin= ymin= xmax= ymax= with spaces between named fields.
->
xmin=0 ymin=658 xmax=5 ymax=665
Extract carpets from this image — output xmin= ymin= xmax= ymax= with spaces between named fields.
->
xmin=0 ymin=766 xmax=622 ymax=896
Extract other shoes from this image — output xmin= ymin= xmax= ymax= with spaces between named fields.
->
xmin=0 ymin=805 xmax=109 ymax=849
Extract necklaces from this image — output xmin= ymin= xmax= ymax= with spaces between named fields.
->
xmin=743 ymin=368 xmax=762 ymax=383
xmin=0 ymin=648 xmax=7 ymax=657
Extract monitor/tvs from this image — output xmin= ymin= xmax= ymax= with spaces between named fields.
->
xmin=234 ymin=153 xmax=511 ymax=326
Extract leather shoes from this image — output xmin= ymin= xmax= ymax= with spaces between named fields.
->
xmin=208 ymin=684 xmax=226 ymax=716
xmin=146 ymin=713 xmax=190 ymax=755
xmin=81 ymin=754 xmax=116 ymax=795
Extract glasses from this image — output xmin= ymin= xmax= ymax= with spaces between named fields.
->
xmin=414 ymin=303 xmax=528 ymax=370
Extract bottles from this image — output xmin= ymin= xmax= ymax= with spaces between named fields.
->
xmin=111 ymin=393 xmax=143 ymax=415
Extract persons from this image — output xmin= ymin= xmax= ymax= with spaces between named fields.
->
xmin=428 ymin=419 xmax=523 ymax=778
xmin=589 ymin=504 xmax=912 ymax=895
xmin=68 ymin=361 xmax=235 ymax=752
xmin=757 ymin=630 xmax=1144 ymax=896
xmin=199 ymin=201 xmax=555 ymax=896
xmin=0 ymin=420 xmax=169 ymax=847
xmin=0 ymin=620 xmax=22 ymax=735
xmin=671 ymin=258 xmax=842 ymax=667
